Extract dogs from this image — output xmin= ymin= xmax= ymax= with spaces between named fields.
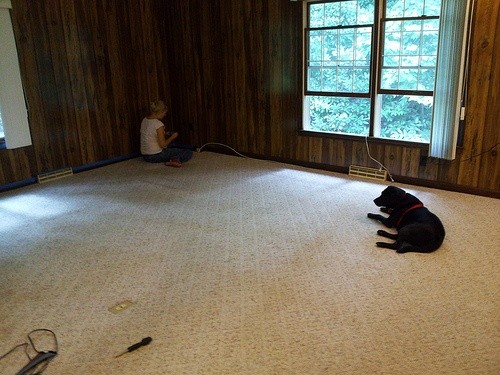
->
xmin=367 ymin=185 xmax=446 ymax=254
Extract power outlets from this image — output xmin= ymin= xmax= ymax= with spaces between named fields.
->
xmin=109 ymin=300 xmax=133 ymax=313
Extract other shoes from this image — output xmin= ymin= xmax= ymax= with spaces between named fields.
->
xmin=165 ymin=159 xmax=182 ymax=167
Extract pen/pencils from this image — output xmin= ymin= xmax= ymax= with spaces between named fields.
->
xmin=113 ymin=337 xmax=152 ymax=358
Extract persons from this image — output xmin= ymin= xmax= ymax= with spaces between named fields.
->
xmin=140 ymin=100 xmax=193 ymax=166
